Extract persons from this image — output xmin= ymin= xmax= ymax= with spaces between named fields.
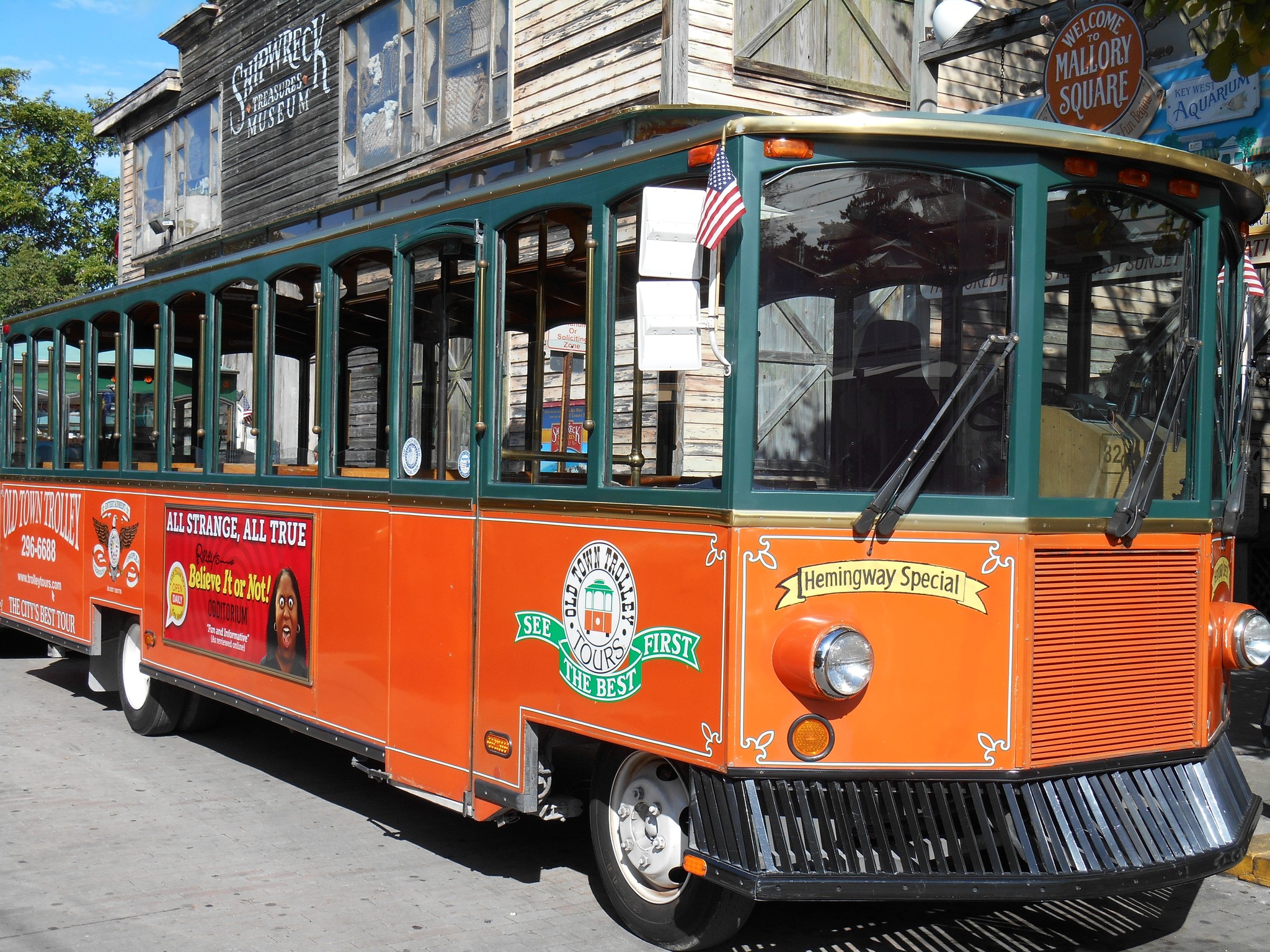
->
xmin=260 ymin=567 xmax=309 ymax=679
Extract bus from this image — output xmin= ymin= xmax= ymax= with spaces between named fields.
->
xmin=3 ymin=97 xmax=1270 ymax=952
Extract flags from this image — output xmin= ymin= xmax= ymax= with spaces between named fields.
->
xmin=1215 ymin=248 xmax=1264 ymax=300
xmin=695 ymin=143 xmax=747 ymax=251
